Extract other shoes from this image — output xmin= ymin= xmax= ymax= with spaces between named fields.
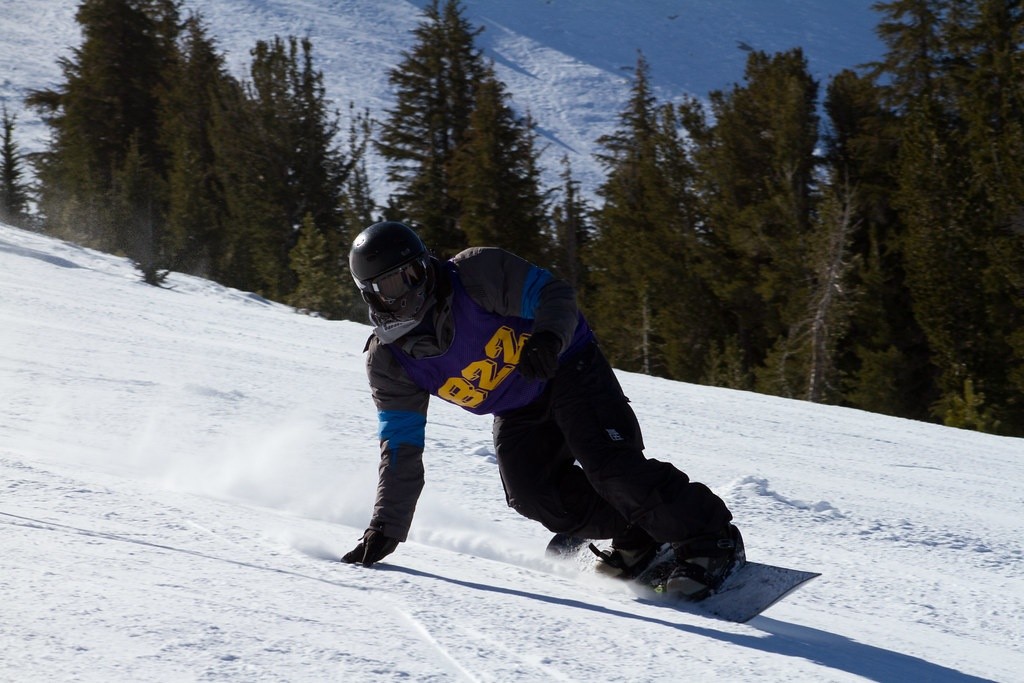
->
xmin=596 ymin=540 xmax=659 ymax=580
xmin=666 ymin=525 xmax=735 ymax=598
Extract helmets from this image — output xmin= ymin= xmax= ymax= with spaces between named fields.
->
xmin=349 ymin=222 xmax=434 ymax=323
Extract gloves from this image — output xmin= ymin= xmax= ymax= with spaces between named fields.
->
xmin=341 ymin=526 xmax=399 ymax=567
xmin=518 ymin=330 xmax=562 ymax=384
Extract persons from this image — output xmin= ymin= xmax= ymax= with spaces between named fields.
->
xmin=340 ymin=221 xmax=748 ymax=602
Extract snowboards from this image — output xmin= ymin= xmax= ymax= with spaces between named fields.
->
xmin=545 ymin=531 xmax=824 ymax=622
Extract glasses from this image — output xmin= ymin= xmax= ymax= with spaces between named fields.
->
xmin=368 ymin=254 xmax=428 ymax=303
